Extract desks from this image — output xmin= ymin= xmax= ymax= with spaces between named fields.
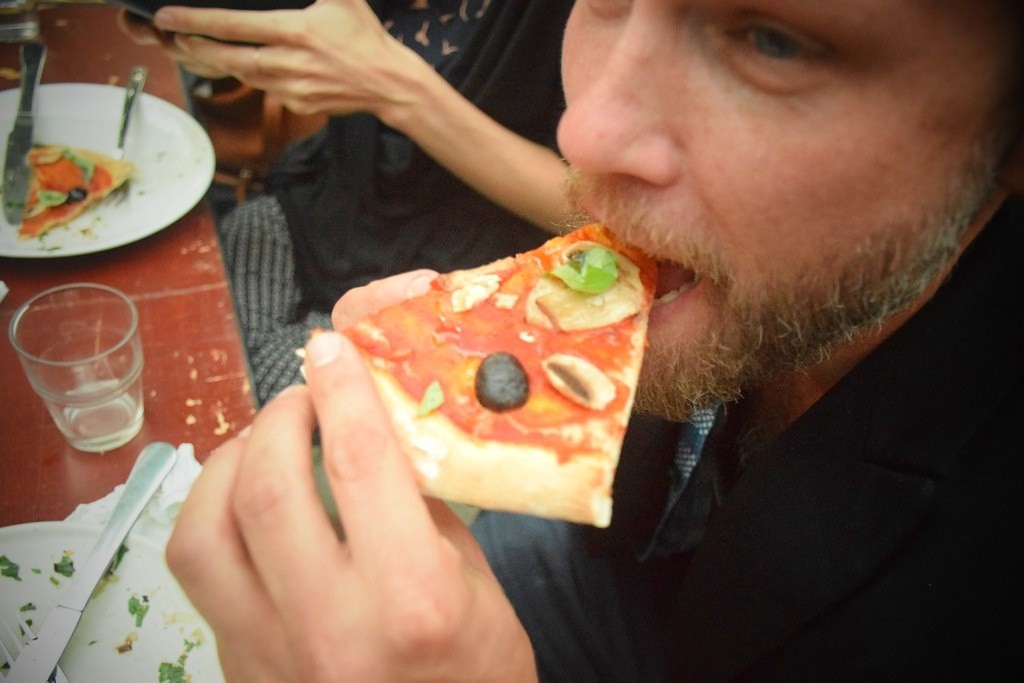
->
xmin=0 ymin=0 xmax=286 ymax=528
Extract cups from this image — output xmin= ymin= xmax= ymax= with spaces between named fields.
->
xmin=8 ymin=281 xmax=147 ymax=453
xmin=0 ymin=0 xmax=41 ymax=44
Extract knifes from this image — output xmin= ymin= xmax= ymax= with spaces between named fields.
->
xmin=0 ymin=42 xmax=47 ymax=226
xmin=0 ymin=440 xmax=180 ymax=683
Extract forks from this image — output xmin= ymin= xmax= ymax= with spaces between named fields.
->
xmin=103 ymin=65 xmax=151 ymax=208
xmin=0 ymin=615 xmax=69 ymax=683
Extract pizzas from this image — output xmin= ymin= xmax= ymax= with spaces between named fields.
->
xmin=296 ymin=218 xmax=659 ymax=529
xmin=17 ymin=144 xmax=137 ymax=240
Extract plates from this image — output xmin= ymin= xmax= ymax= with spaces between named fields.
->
xmin=0 ymin=82 xmax=218 ymax=259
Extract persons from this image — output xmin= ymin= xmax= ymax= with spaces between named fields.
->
xmin=118 ymin=0 xmax=578 ymax=414
xmin=167 ymin=0 xmax=1024 ymax=683
